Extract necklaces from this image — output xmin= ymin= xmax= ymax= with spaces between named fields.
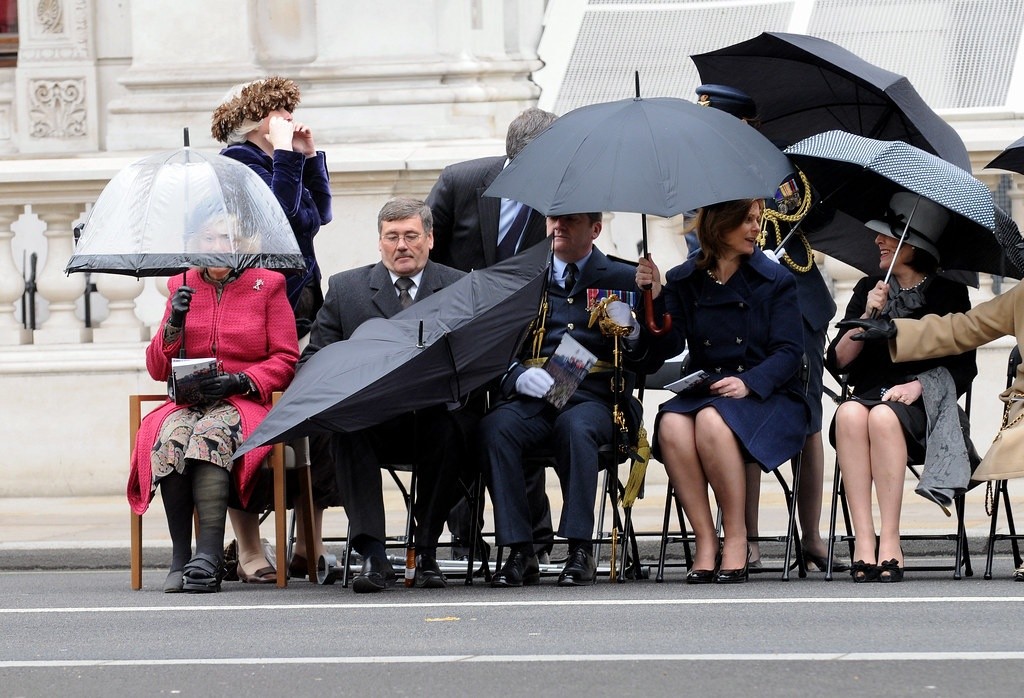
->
xmin=901 ymin=277 xmax=928 ymax=292
xmin=707 ymin=269 xmax=724 ymax=286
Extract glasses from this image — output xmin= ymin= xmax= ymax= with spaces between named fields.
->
xmin=380 ymin=234 xmax=426 ymax=244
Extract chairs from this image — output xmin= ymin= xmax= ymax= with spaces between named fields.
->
xmin=129 ymin=392 xmax=317 ymax=587
xmin=824 ymin=373 xmax=975 ymax=579
xmin=287 ymin=367 xmax=646 ymax=583
xmin=983 ymin=344 xmax=1024 ymax=579
xmin=654 ymin=351 xmax=811 ymax=581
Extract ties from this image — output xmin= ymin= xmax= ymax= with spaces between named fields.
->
xmin=497 ymin=204 xmax=530 ymax=263
xmin=565 ymin=263 xmax=577 ymax=294
xmin=395 ymin=277 xmax=414 ymax=309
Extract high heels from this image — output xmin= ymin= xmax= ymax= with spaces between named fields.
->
xmin=686 ymin=552 xmax=722 ymax=584
xmin=850 ymin=533 xmax=879 ymax=583
xmin=716 ymin=542 xmax=753 ymax=582
xmin=877 ymin=546 xmax=904 ymax=583
xmin=801 ymin=549 xmax=850 ymax=572
xmin=748 ymin=558 xmax=765 ymax=574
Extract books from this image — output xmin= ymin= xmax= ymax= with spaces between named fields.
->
xmin=172 ymin=359 xmax=216 ymax=404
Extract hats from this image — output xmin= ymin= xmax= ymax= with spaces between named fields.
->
xmin=865 ymin=192 xmax=950 ymax=264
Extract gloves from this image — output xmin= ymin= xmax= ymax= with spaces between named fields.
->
xmin=835 ymin=319 xmax=897 ymax=342
xmin=199 ymin=372 xmax=250 ymax=400
xmin=515 ymin=368 xmax=555 ymax=399
xmin=606 ymin=301 xmax=640 ymax=340
xmin=169 ymin=286 xmax=197 ymax=325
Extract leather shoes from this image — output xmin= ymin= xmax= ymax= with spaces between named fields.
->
xmin=558 ymin=543 xmax=597 ymax=586
xmin=491 ymin=551 xmax=541 ymax=587
xmin=538 ymin=551 xmax=550 ymax=564
xmin=414 ymin=554 xmax=447 ymax=588
xmin=453 ymin=539 xmax=490 ymax=561
xmin=353 ymin=555 xmax=398 ymax=593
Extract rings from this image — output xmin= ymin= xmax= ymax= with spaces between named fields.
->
xmin=902 ymin=397 xmax=907 ymax=401
xmin=725 ymin=392 xmax=729 ymax=397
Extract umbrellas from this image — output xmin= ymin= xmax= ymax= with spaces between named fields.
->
xmin=63 ymin=126 xmax=305 ymax=403
xmin=229 ymin=240 xmax=557 ymax=548
xmin=692 ymin=32 xmax=1024 ymax=329
xmin=485 ymin=70 xmax=797 ymax=335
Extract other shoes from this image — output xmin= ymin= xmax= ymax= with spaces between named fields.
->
xmin=236 ymin=557 xmax=277 ymax=584
xmin=1013 ymin=572 xmax=1024 ymax=582
xmin=287 ymin=553 xmax=309 ymax=578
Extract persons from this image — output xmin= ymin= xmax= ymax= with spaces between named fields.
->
xmin=688 ymin=83 xmax=847 ymax=572
xmin=827 ymin=192 xmax=978 ymax=580
xmin=421 ymin=110 xmax=559 ymax=567
xmin=125 ymin=198 xmax=300 ymax=596
xmin=481 ymin=208 xmax=668 ymax=588
xmin=212 ymin=79 xmax=341 ymax=583
xmin=299 ymin=200 xmax=493 ymax=590
xmin=834 ymin=274 xmax=1024 ymax=580
xmin=633 ymin=198 xmax=816 ymax=580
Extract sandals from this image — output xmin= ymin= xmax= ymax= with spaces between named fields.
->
xmin=181 ymin=552 xmax=223 ymax=593
